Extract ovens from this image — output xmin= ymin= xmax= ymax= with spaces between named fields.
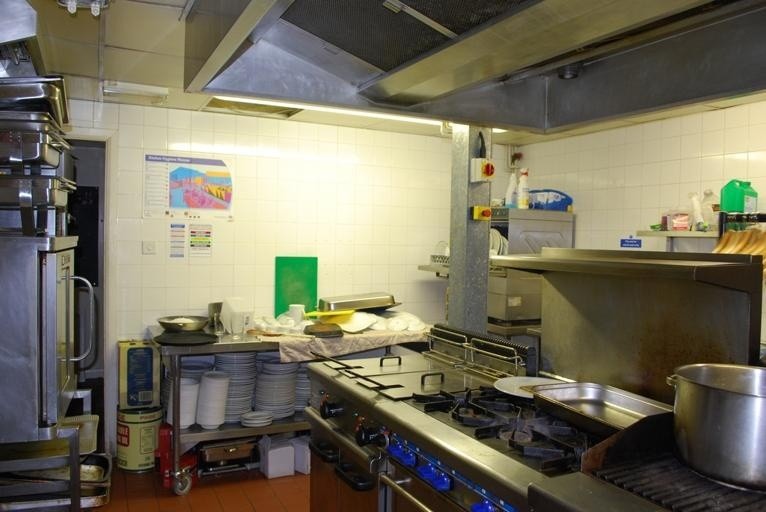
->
xmin=384 ymin=460 xmax=468 ymax=512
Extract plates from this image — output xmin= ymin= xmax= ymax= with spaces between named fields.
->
xmin=495 ymin=376 xmax=568 ymax=400
xmin=341 ymin=312 xmax=425 ymax=333
xmin=161 ymin=352 xmax=311 ymax=431
xmin=435 ymin=228 xmax=509 ymax=266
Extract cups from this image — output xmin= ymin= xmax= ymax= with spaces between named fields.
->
xmin=212 ymin=310 xmax=243 ymax=340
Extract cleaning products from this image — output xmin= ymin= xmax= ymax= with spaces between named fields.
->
xmin=518 ymin=167 xmax=530 ymax=210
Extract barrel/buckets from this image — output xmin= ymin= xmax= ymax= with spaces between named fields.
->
xmin=720 ymin=179 xmax=758 ymax=231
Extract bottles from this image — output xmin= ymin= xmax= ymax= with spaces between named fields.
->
xmin=689 ymin=189 xmax=721 ymax=231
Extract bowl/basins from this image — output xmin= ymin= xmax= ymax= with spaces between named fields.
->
xmin=157 ymin=315 xmax=210 ymax=331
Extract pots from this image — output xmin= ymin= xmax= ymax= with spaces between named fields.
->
xmin=664 ymin=362 xmax=765 ymax=497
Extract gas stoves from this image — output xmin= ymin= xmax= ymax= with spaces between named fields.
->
xmin=401 ymin=384 xmax=598 ymax=478
